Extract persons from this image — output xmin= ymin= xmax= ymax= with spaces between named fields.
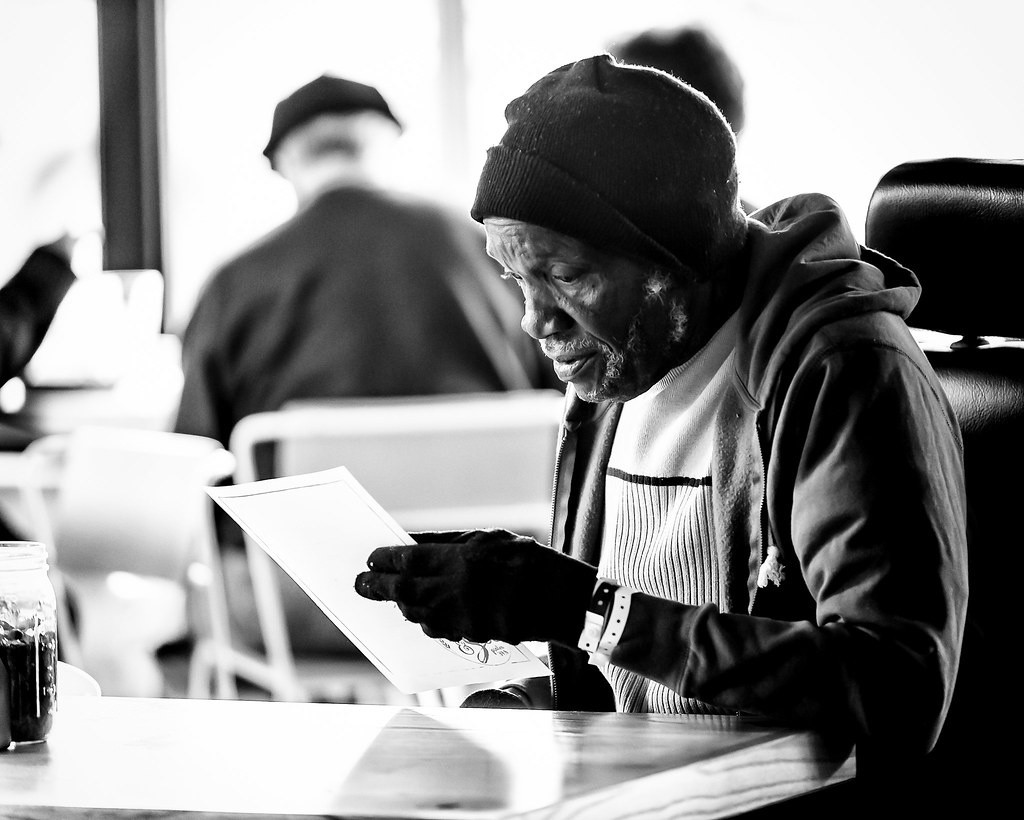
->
xmin=0 ymin=26 xmax=840 ymax=664
xmin=353 ymin=51 xmax=968 ymax=754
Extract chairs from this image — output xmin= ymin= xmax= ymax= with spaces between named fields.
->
xmin=1 ymin=386 xmax=564 ymax=708
xmin=864 ymin=157 xmax=1024 ymax=820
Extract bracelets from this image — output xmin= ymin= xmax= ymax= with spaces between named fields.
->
xmin=576 ymin=564 xmax=630 ymax=666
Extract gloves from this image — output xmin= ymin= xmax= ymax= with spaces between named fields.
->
xmin=459 ymin=689 xmax=528 ymax=709
xmin=354 ymin=529 xmax=598 ymax=652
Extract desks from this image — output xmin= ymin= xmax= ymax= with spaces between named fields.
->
xmin=0 ymin=698 xmax=857 ymax=820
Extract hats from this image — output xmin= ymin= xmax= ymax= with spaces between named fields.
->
xmin=471 ymin=54 xmax=748 ymax=284
xmin=262 ymin=75 xmax=403 ymax=169
xmin=611 ymin=26 xmax=743 ymax=137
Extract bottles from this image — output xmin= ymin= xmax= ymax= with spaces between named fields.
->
xmin=0 ymin=541 xmax=56 ymax=742
